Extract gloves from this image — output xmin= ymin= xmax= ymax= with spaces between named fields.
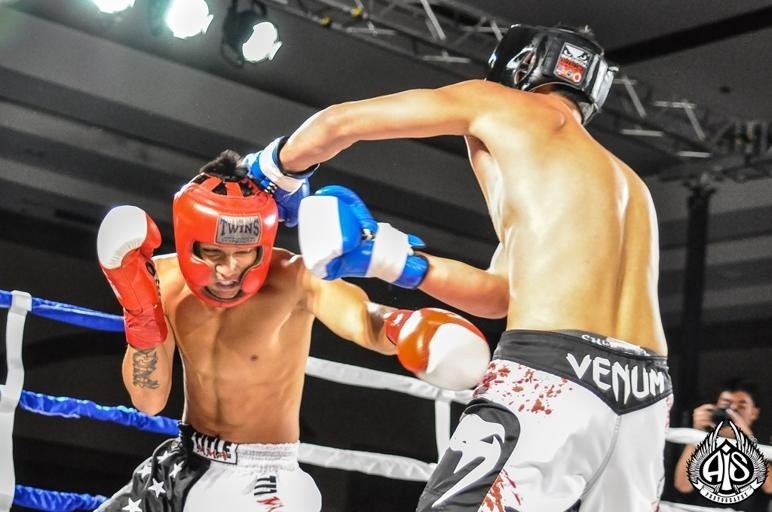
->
xmin=384 ymin=307 xmax=491 ymax=390
xmin=96 ymin=205 xmax=168 ymax=350
xmin=240 ymin=134 xmax=429 ymax=290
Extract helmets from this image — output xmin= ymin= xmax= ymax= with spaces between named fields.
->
xmin=486 ymin=23 xmax=614 ymax=124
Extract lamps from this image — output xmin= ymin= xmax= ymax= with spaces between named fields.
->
xmin=221 ymin=0 xmax=283 ymax=69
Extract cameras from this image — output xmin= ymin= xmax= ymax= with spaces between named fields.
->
xmin=709 ymin=407 xmax=735 ymax=427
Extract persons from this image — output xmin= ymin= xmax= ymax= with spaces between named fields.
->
xmin=239 ymin=21 xmax=678 ymax=512
xmin=91 ymin=150 xmax=490 ymax=512
xmin=671 ymin=377 xmax=772 ymax=512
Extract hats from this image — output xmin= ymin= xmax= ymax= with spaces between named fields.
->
xmin=172 ymin=172 xmax=279 ymax=307
